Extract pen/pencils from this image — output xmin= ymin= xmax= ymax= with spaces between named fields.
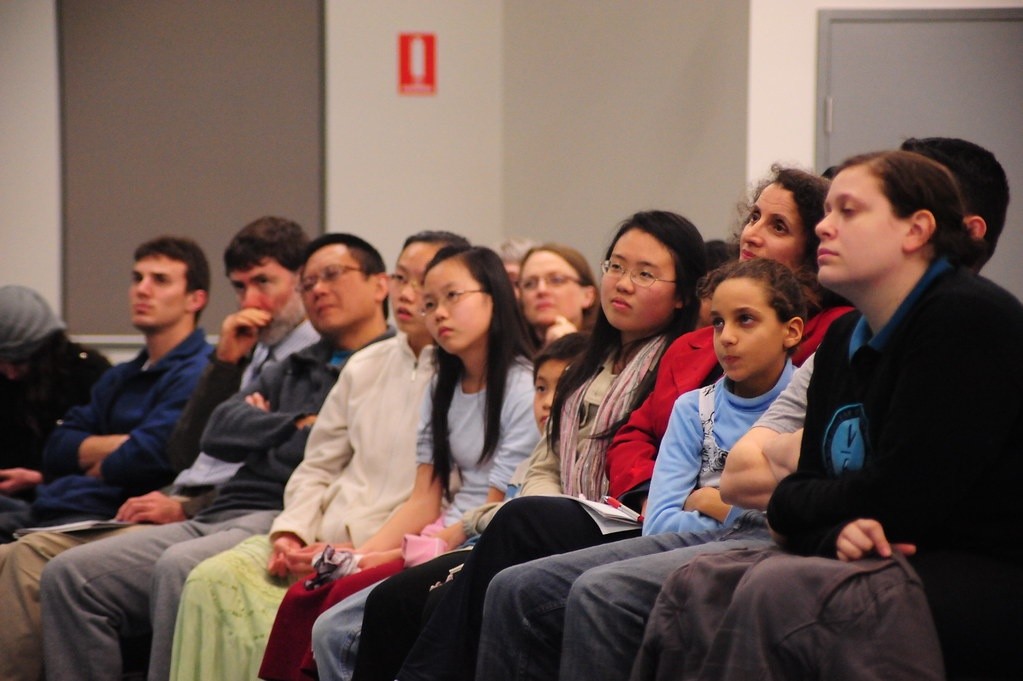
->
xmin=601 ymin=493 xmax=644 ymax=522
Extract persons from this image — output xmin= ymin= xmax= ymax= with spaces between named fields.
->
xmin=350 ymin=169 xmax=856 ymax=681
xmin=475 ymin=135 xmax=1013 ymax=681
xmin=630 ymin=151 xmax=1023 ymax=681
xmin=641 ymin=258 xmax=800 ymax=536
xmin=0 ymin=210 xmax=741 ymax=681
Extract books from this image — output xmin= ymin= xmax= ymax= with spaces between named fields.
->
xmin=16 ymin=517 xmax=133 ymax=533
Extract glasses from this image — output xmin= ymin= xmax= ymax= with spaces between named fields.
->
xmin=514 ymin=275 xmax=582 ymax=290
xmin=600 ymin=258 xmax=677 ymax=288
xmin=420 ymin=288 xmax=485 ymax=317
xmin=295 ymin=265 xmax=366 ymax=300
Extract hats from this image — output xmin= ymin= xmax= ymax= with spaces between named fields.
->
xmin=0 ymin=284 xmax=68 ymax=359
